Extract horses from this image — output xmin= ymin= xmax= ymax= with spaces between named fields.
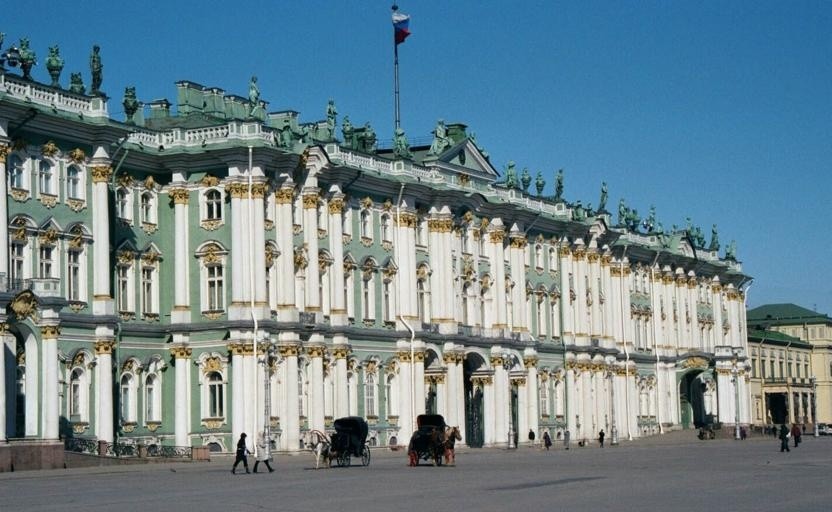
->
xmin=433 ymin=426 xmax=462 ymax=466
xmin=305 ymin=429 xmax=338 ymax=470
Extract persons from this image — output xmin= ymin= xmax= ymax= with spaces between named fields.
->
xmin=90 ymin=45 xmax=103 ymax=91
xmin=740 ymin=424 xmax=806 ymax=452
xmin=506 ymin=160 xmax=737 ymax=259
xmin=528 ymin=429 xmax=535 ymax=447
xmin=233 ymin=433 xmax=252 ymax=474
xmin=324 ymin=99 xmax=455 ymax=155
xmin=543 ymin=432 xmax=552 ymax=450
xmin=249 ymin=76 xmax=259 ymax=107
xmin=408 ymin=431 xmax=420 ymax=467
xmin=599 ymin=428 xmax=604 ymax=448
xmin=563 ymin=429 xmax=570 ymax=450
xmin=253 ymin=431 xmax=275 ymax=473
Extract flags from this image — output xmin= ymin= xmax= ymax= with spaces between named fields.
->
xmin=392 ymin=12 xmax=411 ymax=44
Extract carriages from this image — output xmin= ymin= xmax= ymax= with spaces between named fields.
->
xmin=298 ymin=416 xmax=371 ymax=470
xmin=407 ymin=414 xmax=462 ymax=467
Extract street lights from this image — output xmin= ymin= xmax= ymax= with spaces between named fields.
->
xmin=809 ymin=376 xmax=820 ymax=437
xmin=730 ymin=363 xmax=741 ymax=439
xmin=605 ymin=359 xmax=619 ymax=445
xmin=256 ymin=332 xmax=278 ymax=463
